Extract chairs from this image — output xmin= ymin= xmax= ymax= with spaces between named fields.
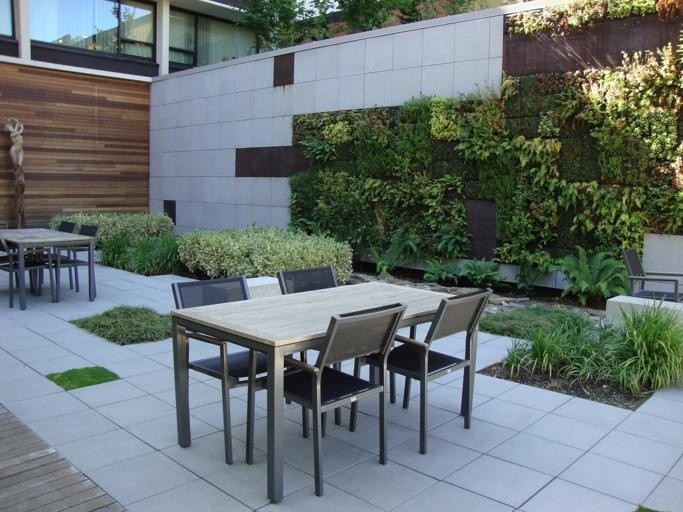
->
xmin=171 ymin=274 xmax=267 ymax=464
xmin=623 ymin=248 xmax=682 ymax=303
xmin=277 ymin=263 xmax=342 ymax=425
xmin=246 ymin=302 xmax=409 ymax=496
xmin=348 ymin=287 xmax=494 ymax=454
xmin=0 ymin=220 xmax=100 ymax=310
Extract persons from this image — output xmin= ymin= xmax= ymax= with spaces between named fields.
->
xmin=4 ymin=117 xmax=24 ymax=166
